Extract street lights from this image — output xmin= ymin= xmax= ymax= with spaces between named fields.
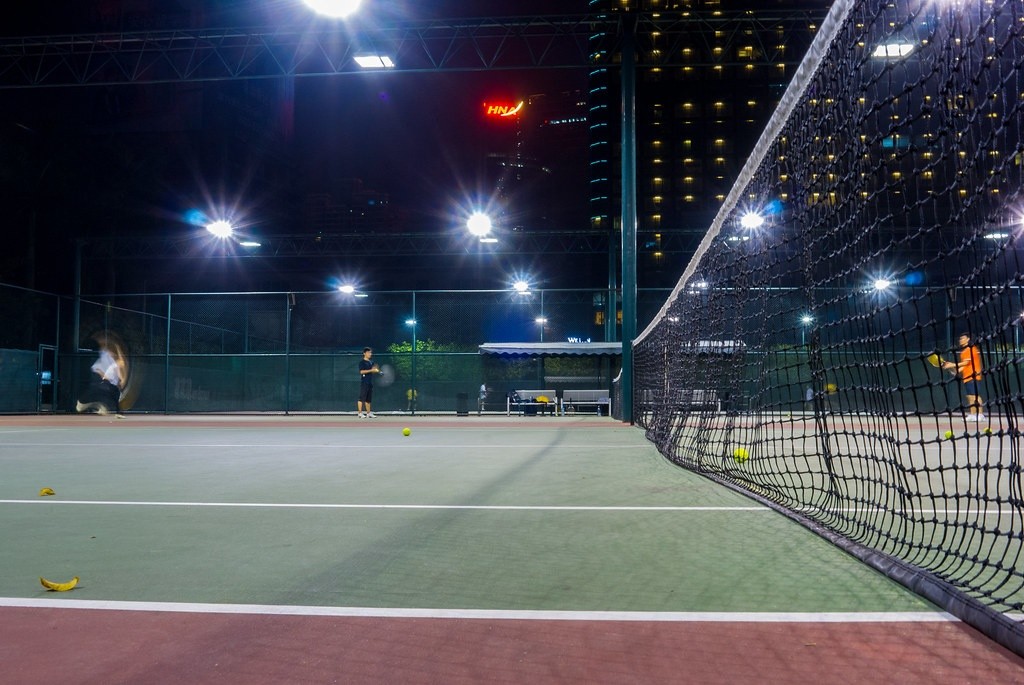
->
xmin=207 ymin=221 xmax=292 ymax=414
xmin=467 ymin=213 xmax=619 ymax=417
xmin=302 ymin=0 xmax=639 ymax=421
xmin=405 ymin=318 xmax=418 ymax=415
xmin=514 ymin=281 xmax=545 ymax=417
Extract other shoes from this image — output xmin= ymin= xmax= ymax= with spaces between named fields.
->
xmin=961 ymin=413 xmax=985 ymax=421
xmin=367 ymin=412 xmax=377 ymax=418
xmin=358 ymin=413 xmax=366 ymax=418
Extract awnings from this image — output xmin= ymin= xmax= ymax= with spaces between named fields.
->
xmin=478 ymin=341 xmax=748 ymax=355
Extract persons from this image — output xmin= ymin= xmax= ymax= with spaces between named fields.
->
xmin=479 ymin=382 xmax=487 ymax=411
xmin=943 ymin=332 xmax=988 ymax=422
xmin=407 ymin=385 xmax=418 ymax=411
xmin=805 ymin=384 xmax=814 ymax=410
xmin=358 ymin=346 xmax=382 ymax=418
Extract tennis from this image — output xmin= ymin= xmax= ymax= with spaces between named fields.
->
xmin=827 ymin=383 xmax=836 ymax=392
xmin=402 ymin=428 xmax=411 ymax=435
xmin=734 ymin=447 xmax=749 ymax=463
xmin=984 ymin=428 xmax=993 ymax=433
xmin=945 ymin=431 xmax=953 ymax=439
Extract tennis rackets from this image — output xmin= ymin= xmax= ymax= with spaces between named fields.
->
xmin=928 ymin=353 xmax=950 ymax=370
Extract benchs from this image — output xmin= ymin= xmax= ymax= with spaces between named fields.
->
xmin=638 ymin=389 xmax=721 ymax=414
xmin=560 ymin=389 xmax=612 ymax=417
xmin=506 ymin=390 xmax=558 ymax=418
